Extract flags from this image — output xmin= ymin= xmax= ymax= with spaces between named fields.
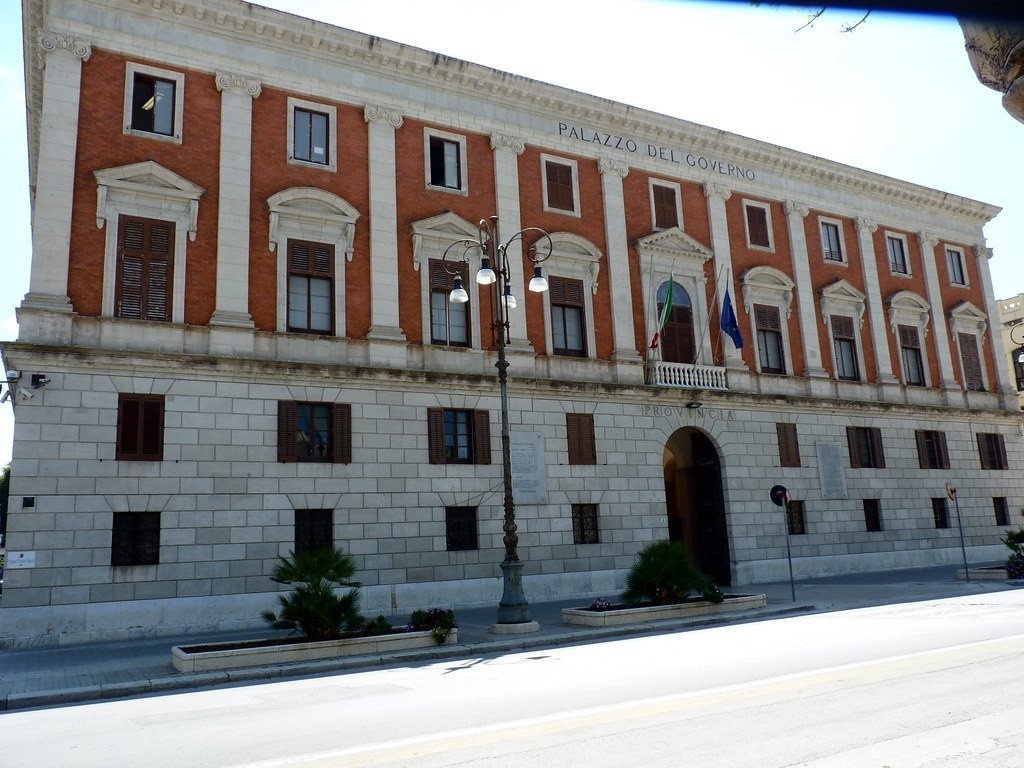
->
xmin=720 ymin=283 xmax=744 ymax=349
xmin=649 ymin=270 xmax=673 ymax=350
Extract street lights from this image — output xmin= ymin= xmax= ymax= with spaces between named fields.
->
xmin=443 ymin=215 xmax=553 ymax=634
xmin=1010 ymin=322 xmax=1024 ymax=426
